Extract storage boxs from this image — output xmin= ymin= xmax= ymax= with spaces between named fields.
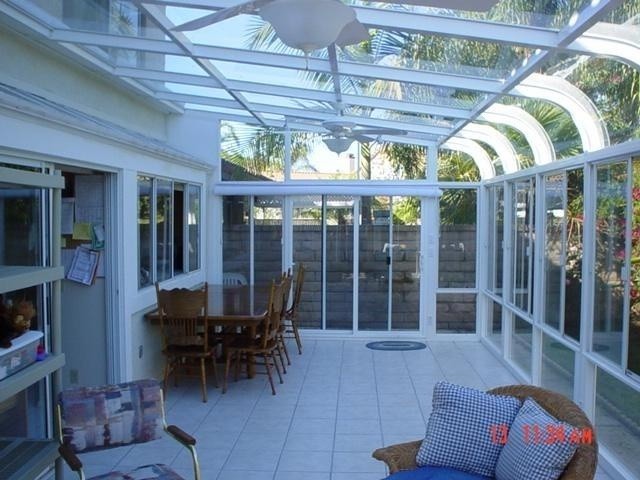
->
xmin=0 ymin=328 xmax=45 ymax=383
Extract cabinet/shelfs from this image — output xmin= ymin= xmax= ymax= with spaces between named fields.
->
xmin=0 ymin=263 xmax=67 ymax=479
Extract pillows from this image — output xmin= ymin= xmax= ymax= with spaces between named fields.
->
xmin=415 ymin=378 xmax=583 ymax=480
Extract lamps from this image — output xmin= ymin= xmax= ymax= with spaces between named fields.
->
xmin=258 ymin=0 xmax=360 ymax=55
xmin=321 ymin=121 xmax=357 ymax=158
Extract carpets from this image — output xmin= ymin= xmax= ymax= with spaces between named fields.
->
xmin=365 ymin=340 xmax=427 ymax=352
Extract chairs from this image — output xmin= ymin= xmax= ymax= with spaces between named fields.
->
xmin=152 ymin=259 xmax=305 ymax=404
xmin=371 ymin=382 xmax=599 ymax=480
xmin=51 ymin=377 xmax=201 ymax=480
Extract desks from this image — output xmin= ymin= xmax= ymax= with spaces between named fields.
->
xmin=146 ymin=284 xmax=275 ymax=378
